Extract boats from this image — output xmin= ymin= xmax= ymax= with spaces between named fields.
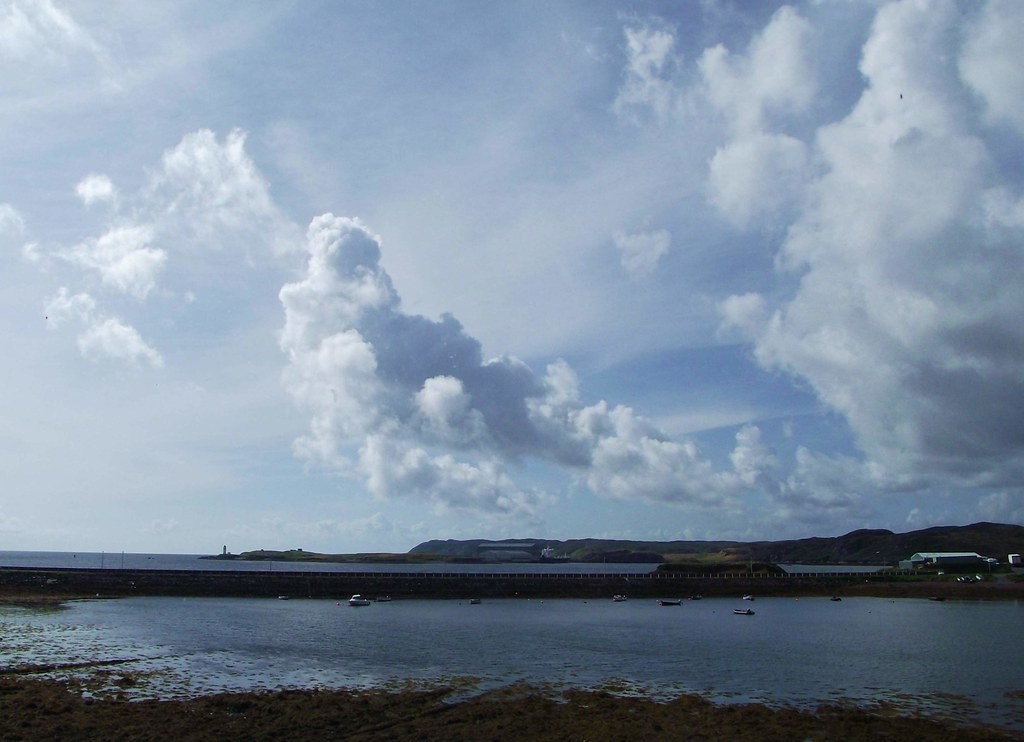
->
xmin=469 ymin=599 xmax=481 ymax=604
xmin=278 ymin=595 xmax=289 ymax=600
xmin=377 ymin=596 xmax=391 ymax=601
xmin=688 ymin=593 xmax=703 ymax=599
xmin=732 ymin=608 xmax=755 ymax=615
xmin=743 ymin=595 xmax=753 ymax=600
xmin=928 ymin=596 xmax=945 ymax=601
xmin=623 ymin=595 xmax=627 ymax=600
xmin=657 ymin=600 xmax=682 ymax=606
xmin=613 ymin=594 xmax=621 ymax=601
xmin=539 ymin=546 xmax=570 ymax=563
xmin=830 ymin=596 xmax=841 ymax=601
xmin=349 ymin=595 xmax=369 ymax=605
